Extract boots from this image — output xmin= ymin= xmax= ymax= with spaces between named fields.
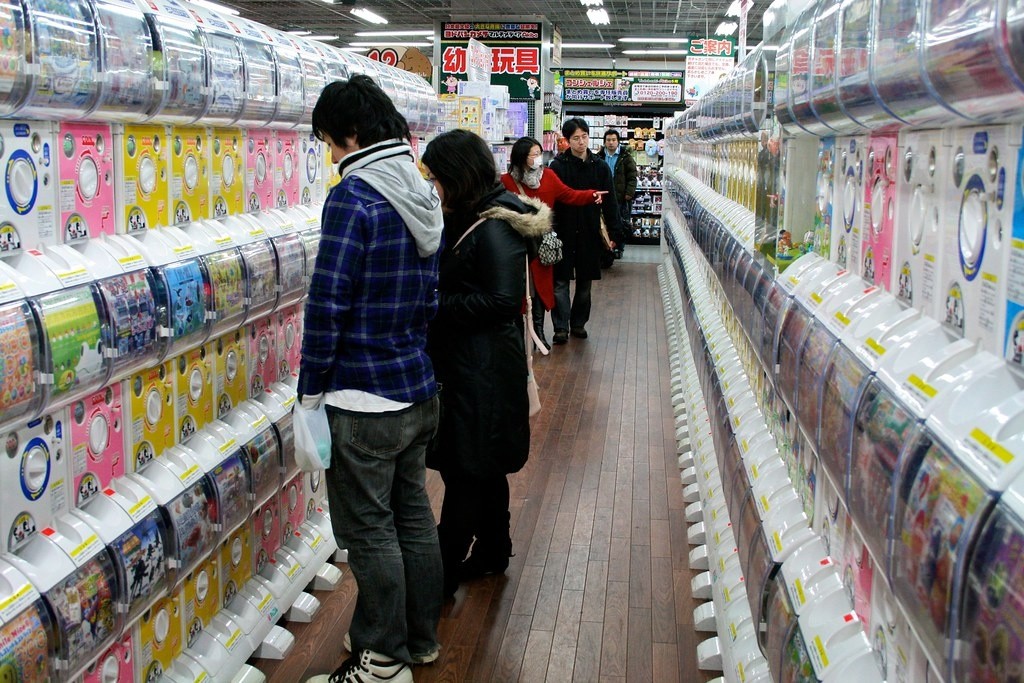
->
xmin=532 ymin=309 xmax=551 ymax=350
xmin=459 ymin=509 xmax=512 ymax=581
xmin=434 ymin=523 xmax=474 ymax=595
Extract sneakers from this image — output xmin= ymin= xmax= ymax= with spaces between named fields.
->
xmin=305 ymin=649 xmax=413 ymax=683
xmin=344 ymin=631 xmax=439 ymax=663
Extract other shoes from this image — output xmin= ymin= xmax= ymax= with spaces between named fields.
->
xmin=571 ymin=326 xmax=588 ymax=339
xmin=553 ymin=330 xmax=569 ymax=342
xmin=615 ymin=250 xmax=623 ymax=259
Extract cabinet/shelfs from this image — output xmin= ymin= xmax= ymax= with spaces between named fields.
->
xmin=565 ymin=114 xmax=667 ymax=245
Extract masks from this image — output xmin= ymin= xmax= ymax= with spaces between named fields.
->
xmin=526 ymin=154 xmax=543 ymax=169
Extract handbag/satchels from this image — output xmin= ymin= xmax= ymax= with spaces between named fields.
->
xmin=525 ymin=353 xmax=542 ymax=417
xmin=538 ymin=232 xmax=563 ymax=266
xmin=293 ymin=395 xmax=332 ymax=470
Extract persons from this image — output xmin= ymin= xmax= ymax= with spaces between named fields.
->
xmin=291 ymin=74 xmax=443 ymax=683
xmin=499 ymin=117 xmax=637 ymax=351
xmin=422 ymin=129 xmax=554 ymax=596
